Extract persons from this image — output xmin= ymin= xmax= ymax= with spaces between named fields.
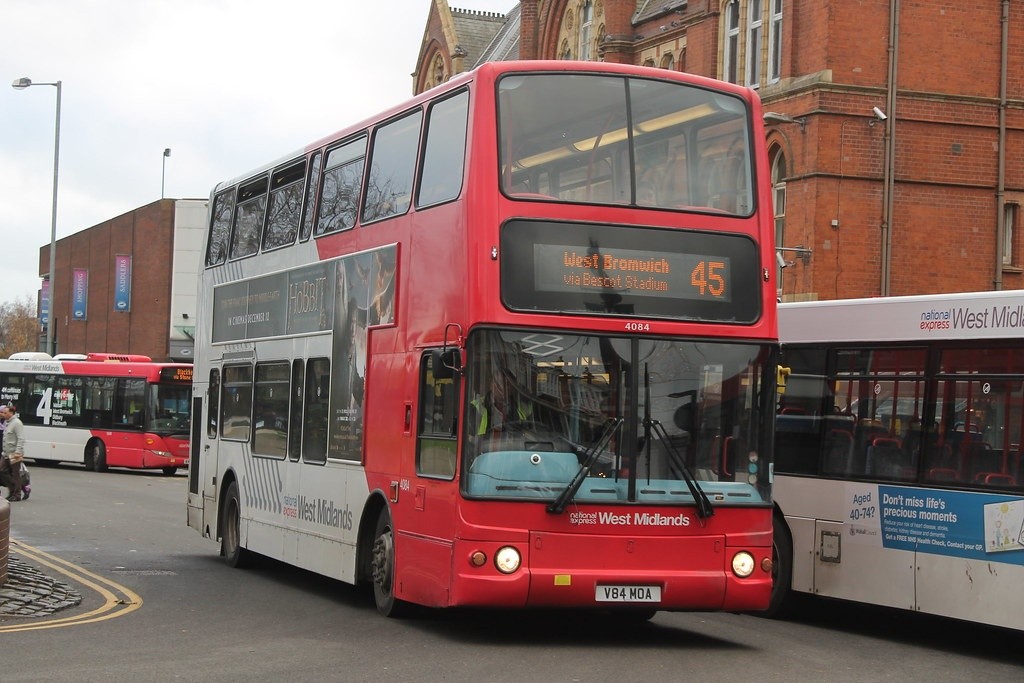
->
xmin=467 ymin=368 xmax=535 ymax=448
xmin=0 ymin=405 xmax=33 ymax=502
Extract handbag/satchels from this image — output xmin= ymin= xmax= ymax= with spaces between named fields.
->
xmin=19 ymin=463 xmax=30 ymax=486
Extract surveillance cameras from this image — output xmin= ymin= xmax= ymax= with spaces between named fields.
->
xmin=776 ymin=252 xmax=787 ymax=268
xmin=873 ymin=107 xmax=887 ymax=120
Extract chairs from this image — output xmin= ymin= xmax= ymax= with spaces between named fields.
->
xmin=226 ymin=416 xmax=293 ymax=458
xmin=774 ymin=405 xmax=1024 ymax=490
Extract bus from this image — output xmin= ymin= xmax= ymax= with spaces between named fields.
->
xmin=183 ymin=60 xmax=791 ymax=631
xmin=0 ymin=351 xmax=193 ymax=477
xmin=691 ymin=288 xmax=1024 ymax=632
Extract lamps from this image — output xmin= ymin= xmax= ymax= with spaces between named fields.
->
xmin=763 ymin=112 xmax=806 ymax=131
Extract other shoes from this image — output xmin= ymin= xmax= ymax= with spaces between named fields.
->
xmin=22 ymin=488 xmax=31 ymax=500
xmin=5 ymin=495 xmax=21 ymax=501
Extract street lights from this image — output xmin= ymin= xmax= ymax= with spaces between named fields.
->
xmin=11 ymin=77 xmax=62 ymax=358
xmin=161 ymin=146 xmax=173 ymax=200
